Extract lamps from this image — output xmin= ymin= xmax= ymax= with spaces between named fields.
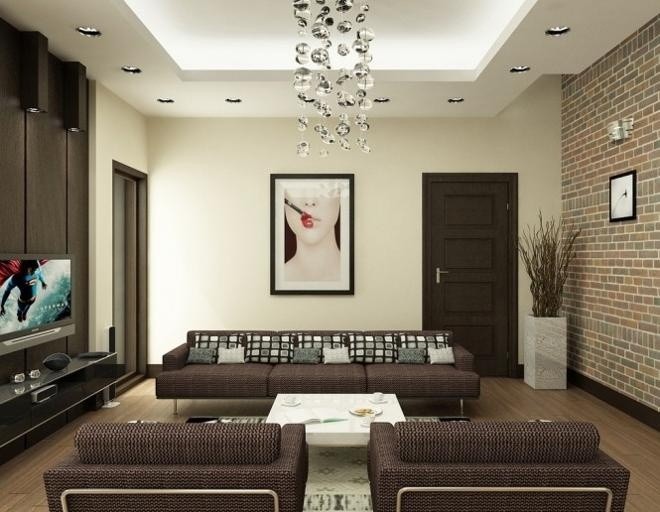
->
xmin=19 ymin=30 xmax=51 ymax=116
xmin=290 ymin=0 xmax=378 ymax=164
xmin=59 ymin=59 xmax=90 ymax=134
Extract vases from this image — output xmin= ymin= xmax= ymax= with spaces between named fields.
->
xmin=525 ymin=315 xmax=568 ymax=389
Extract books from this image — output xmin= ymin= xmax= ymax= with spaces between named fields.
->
xmin=283 ymin=404 xmax=350 ymax=424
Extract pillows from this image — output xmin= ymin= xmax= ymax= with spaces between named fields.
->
xmin=188 ymin=332 xmax=461 ymax=364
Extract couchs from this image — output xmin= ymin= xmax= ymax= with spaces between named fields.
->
xmin=154 ymin=327 xmax=483 ymax=415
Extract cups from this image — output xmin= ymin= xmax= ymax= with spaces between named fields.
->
xmin=372 ymin=392 xmax=384 ymax=401
xmin=284 ymin=396 xmax=296 ymax=404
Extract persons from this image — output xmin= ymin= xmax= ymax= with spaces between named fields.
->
xmin=284 ymin=181 xmax=341 ymax=282
xmin=0 ymin=259 xmax=49 ymax=322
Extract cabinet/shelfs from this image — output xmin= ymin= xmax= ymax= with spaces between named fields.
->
xmin=0 ymin=352 xmax=128 ymax=464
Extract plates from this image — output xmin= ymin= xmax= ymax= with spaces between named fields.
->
xmin=349 ymin=406 xmax=383 ymax=416
xmin=282 ymin=402 xmax=300 ymax=407
xmin=368 ymin=398 xmax=387 ymax=404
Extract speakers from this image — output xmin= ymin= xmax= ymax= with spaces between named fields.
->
xmin=101 ymin=326 xmax=120 ymax=409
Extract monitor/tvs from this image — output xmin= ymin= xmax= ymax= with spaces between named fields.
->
xmin=0 ymin=253 xmax=75 ymax=356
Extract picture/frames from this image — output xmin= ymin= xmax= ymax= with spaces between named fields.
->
xmin=608 ymin=170 xmax=637 ymax=222
xmin=269 ymin=174 xmax=357 ymax=295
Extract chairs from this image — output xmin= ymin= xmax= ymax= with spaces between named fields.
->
xmin=43 ymin=423 xmax=307 ymax=510
xmin=365 ymin=418 xmax=633 ymax=509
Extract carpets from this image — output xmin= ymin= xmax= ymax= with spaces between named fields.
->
xmin=181 ymin=410 xmax=478 ymax=512
